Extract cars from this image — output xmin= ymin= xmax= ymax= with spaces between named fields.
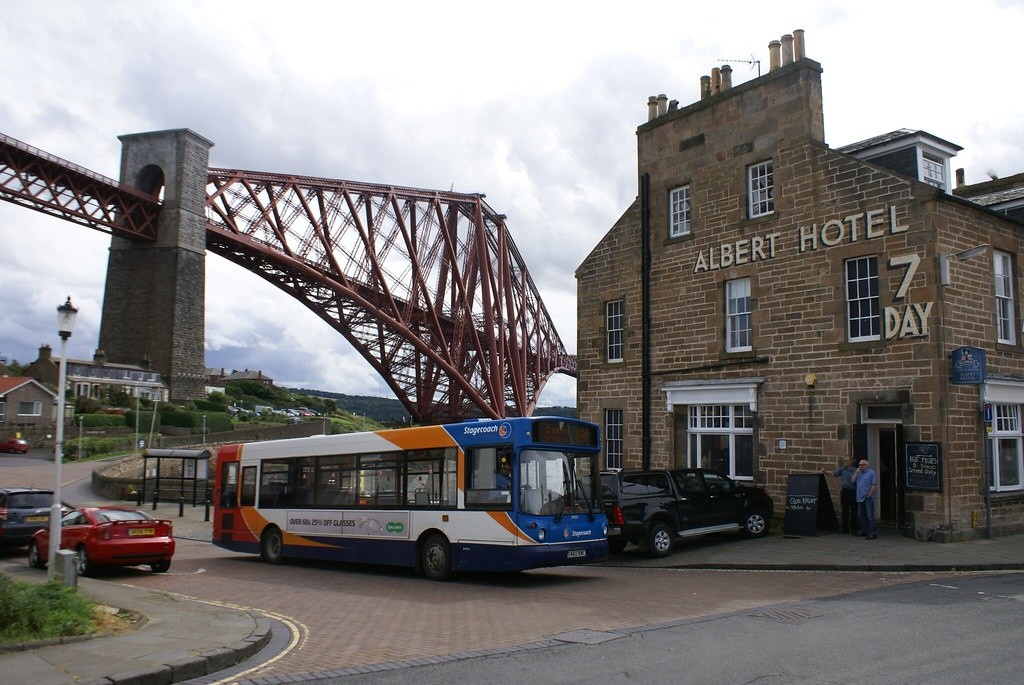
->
xmin=224 ymin=406 xmax=323 ymax=424
xmin=28 ymin=506 xmax=176 ymax=577
xmin=0 ymin=438 xmax=29 ymax=454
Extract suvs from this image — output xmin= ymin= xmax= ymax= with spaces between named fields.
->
xmin=579 ymin=468 xmax=774 ymax=559
xmin=0 ymin=488 xmax=77 ymax=557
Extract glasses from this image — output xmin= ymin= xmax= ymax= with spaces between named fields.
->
xmin=859 ymin=464 xmax=867 ymax=466
xmin=843 ymin=459 xmax=850 ymax=462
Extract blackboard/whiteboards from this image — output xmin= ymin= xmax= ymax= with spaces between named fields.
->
xmin=782 ymin=473 xmax=840 ymax=537
xmin=904 ymin=442 xmax=941 ymax=492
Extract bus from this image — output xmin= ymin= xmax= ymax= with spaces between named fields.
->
xmin=212 ymin=416 xmax=609 ymax=583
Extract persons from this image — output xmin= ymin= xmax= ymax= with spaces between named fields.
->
xmin=851 ymin=459 xmax=877 ymax=540
xmin=821 ymin=455 xmax=857 ymax=537
xmin=490 ymin=463 xmax=532 ymax=498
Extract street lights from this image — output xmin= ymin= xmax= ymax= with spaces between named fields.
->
xmin=46 ymin=294 xmax=79 ymax=578
xmin=78 ymin=414 xmax=84 ymax=460
xmin=363 ymin=413 xmax=366 ymax=431
xmin=202 ymin=415 xmax=206 ymax=445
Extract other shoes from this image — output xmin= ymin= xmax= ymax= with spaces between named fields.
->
xmin=840 ymin=530 xmax=849 ymax=534
xmin=857 ymin=534 xmax=867 ymax=537
xmin=865 ymin=536 xmax=877 ymax=540
xmin=851 ymin=531 xmax=856 ymax=536
